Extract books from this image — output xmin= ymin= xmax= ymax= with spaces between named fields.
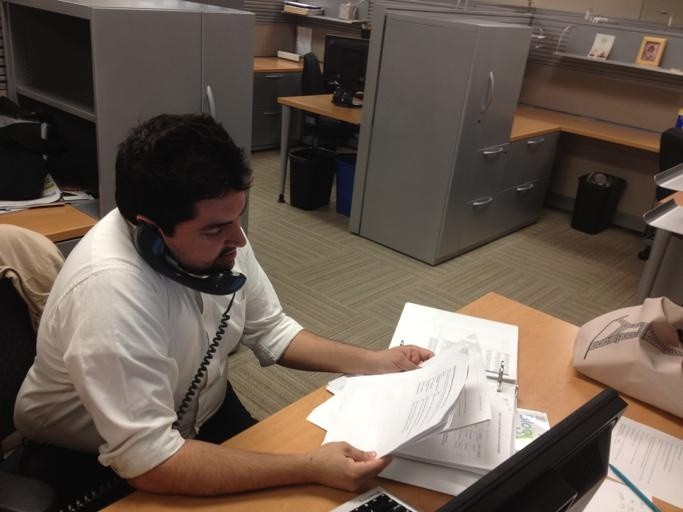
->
xmin=0 ymin=173 xmax=65 ymax=208
xmin=284 ymin=1 xmax=324 ymax=16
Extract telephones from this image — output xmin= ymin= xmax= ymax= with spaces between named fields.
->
xmin=129 ymin=219 xmax=249 ymax=296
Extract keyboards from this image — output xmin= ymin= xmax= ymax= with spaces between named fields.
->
xmin=324 ymin=483 xmax=418 ymax=512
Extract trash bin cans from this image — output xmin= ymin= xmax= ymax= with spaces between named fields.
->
xmin=338 ymin=150 xmax=357 ymax=217
xmin=568 ymin=172 xmax=629 ymax=235
xmin=283 ymin=143 xmax=339 ymax=212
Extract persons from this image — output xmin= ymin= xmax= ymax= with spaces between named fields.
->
xmin=0 ymin=224 xmax=65 ymax=335
xmin=12 ymin=113 xmax=435 ymax=512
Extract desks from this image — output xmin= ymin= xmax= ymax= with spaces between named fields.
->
xmin=96 ymin=291 xmax=683 ymax=511
xmin=632 ymin=162 xmax=682 ymax=305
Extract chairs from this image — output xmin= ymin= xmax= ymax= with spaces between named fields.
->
xmin=0 ymin=275 xmax=56 ymax=510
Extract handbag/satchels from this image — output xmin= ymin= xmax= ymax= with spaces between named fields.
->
xmin=573 ymin=296 xmax=682 ymax=420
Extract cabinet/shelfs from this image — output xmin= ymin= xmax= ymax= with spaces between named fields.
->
xmin=0 ymin=1 xmax=683 ymax=266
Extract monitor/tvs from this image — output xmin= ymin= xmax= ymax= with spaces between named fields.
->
xmin=437 ymin=388 xmax=628 ymax=511
xmin=321 ymin=33 xmax=371 ymax=109
